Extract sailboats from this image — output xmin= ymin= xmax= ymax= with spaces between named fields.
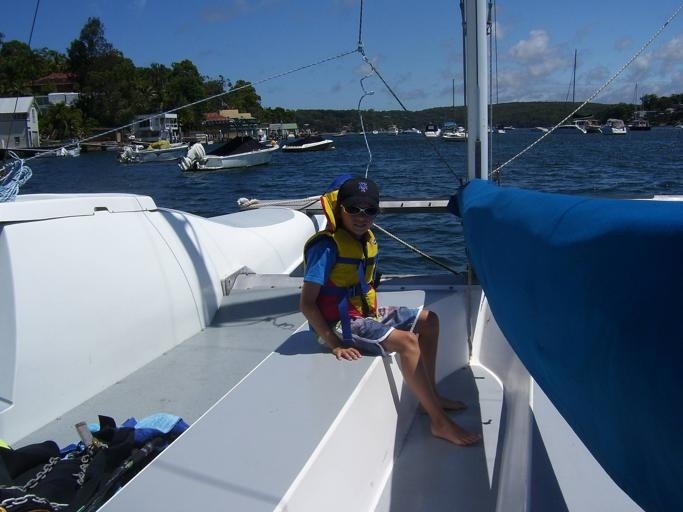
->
xmin=442 ymin=77 xmax=470 ymax=143
xmin=628 ymin=80 xmax=653 ymax=131
xmin=562 ymin=48 xmax=601 ymax=134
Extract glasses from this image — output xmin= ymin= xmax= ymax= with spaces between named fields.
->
xmin=340 ymin=204 xmax=378 ymax=217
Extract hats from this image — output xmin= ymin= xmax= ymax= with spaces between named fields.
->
xmin=337 ymin=178 xmax=379 ymax=208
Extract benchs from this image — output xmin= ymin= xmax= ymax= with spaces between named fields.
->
xmin=95 ymin=289 xmax=426 ymax=512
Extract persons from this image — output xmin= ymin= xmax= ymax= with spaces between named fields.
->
xmin=300 ymin=177 xmax=482 ymax=446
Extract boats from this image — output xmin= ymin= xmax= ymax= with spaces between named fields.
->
xmin=529 ymin=119 xmax=630 ymax=137
xmin=1 ymin=1 xmax=682 ymax=512
xmin=335 ymin=122 xmax=515 ymax=137
xmin=114 ymin=126 xmax=337 ymax=173
